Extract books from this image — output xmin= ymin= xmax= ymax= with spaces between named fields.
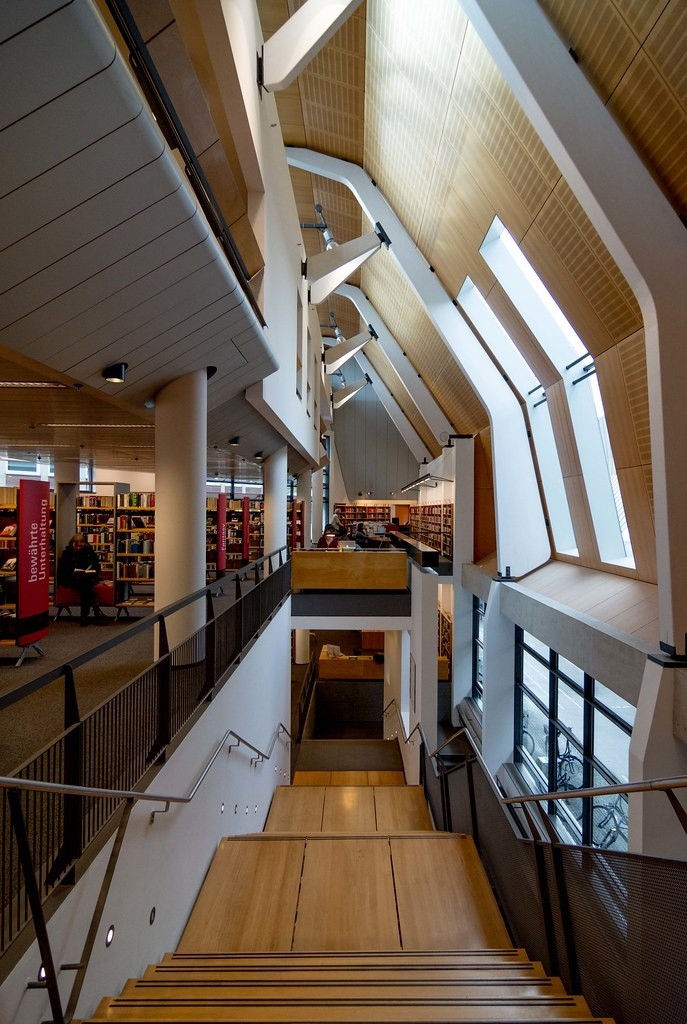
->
xmin=438 ymin=611 xmax=450 ymax=657
xmin=337 ymin=506 xmax=390 ymax=533
xmin=74 ymin=564 xmax=96 ymax=574
xmin=206 ymin=497 xmax=301 ymax=582
xmin=49 ymin=492 xmax=155 ymax=606
xmin=349 ymin=657 xmax=357 ymax=661
xmin=0 ymin=523 xmax=17 ymax=536
xmin=410 ymin=508 xmax=452 ymax=555
xmin=1 ymin=558 xmax=16 ymax=571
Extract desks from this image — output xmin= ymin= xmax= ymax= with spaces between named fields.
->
xmin=390 ymin=530 xmax=440 ymax=568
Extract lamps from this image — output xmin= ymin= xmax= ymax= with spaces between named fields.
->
xmin=105 ymin=364 xmax=126 ymax=383
xmin=229 ymin=437 xmax=239 ymax=446
xmin=329 ymin=368 xmax=349 ymax=389
xmin=319 ymin=312 xmax=346 ymax=345
xmin=300 ymin=204 xmax=338 ymax=251
xmin=254 ymin=452 xmax=262 ymax=460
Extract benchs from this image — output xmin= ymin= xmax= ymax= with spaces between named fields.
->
xmin=53 ymin=571 xmax=130 ymax=621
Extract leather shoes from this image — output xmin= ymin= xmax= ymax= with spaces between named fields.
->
xmin=80 ymin=615 xmax=89 ymax=627
xmin=94 ymin=609 xmax=107 ymax=619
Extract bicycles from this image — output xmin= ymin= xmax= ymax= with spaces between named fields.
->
xmin=522 ymin=713 xmax=630 ymax=852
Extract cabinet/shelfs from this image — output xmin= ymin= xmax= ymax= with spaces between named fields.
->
xmin=334 ymin=504 xmax=391 ymax=534
xmin=438 ymin=608 xmax=453 ymax=674
xmin=407 ymin=503 xmax=453 ymax=561
xmin=0 ymin=479 xmax=305 ymax=669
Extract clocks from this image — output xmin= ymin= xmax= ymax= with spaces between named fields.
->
xmin=439 ymin=432 xmax=450 ymax=442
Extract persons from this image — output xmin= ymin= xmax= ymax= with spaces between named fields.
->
xmin=57 ymin=533 xmax=110 ymax=627
xmin=317 ymin=528 xmax=339 ymax=551
xmin=355 ymin=523 xmax=368 ymax=548
xmin=330 ymin=509 xmax=344 ymax=534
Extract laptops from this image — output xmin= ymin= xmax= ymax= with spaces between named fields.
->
xmin=339 ymin=541 xmax=355 ymax=552
